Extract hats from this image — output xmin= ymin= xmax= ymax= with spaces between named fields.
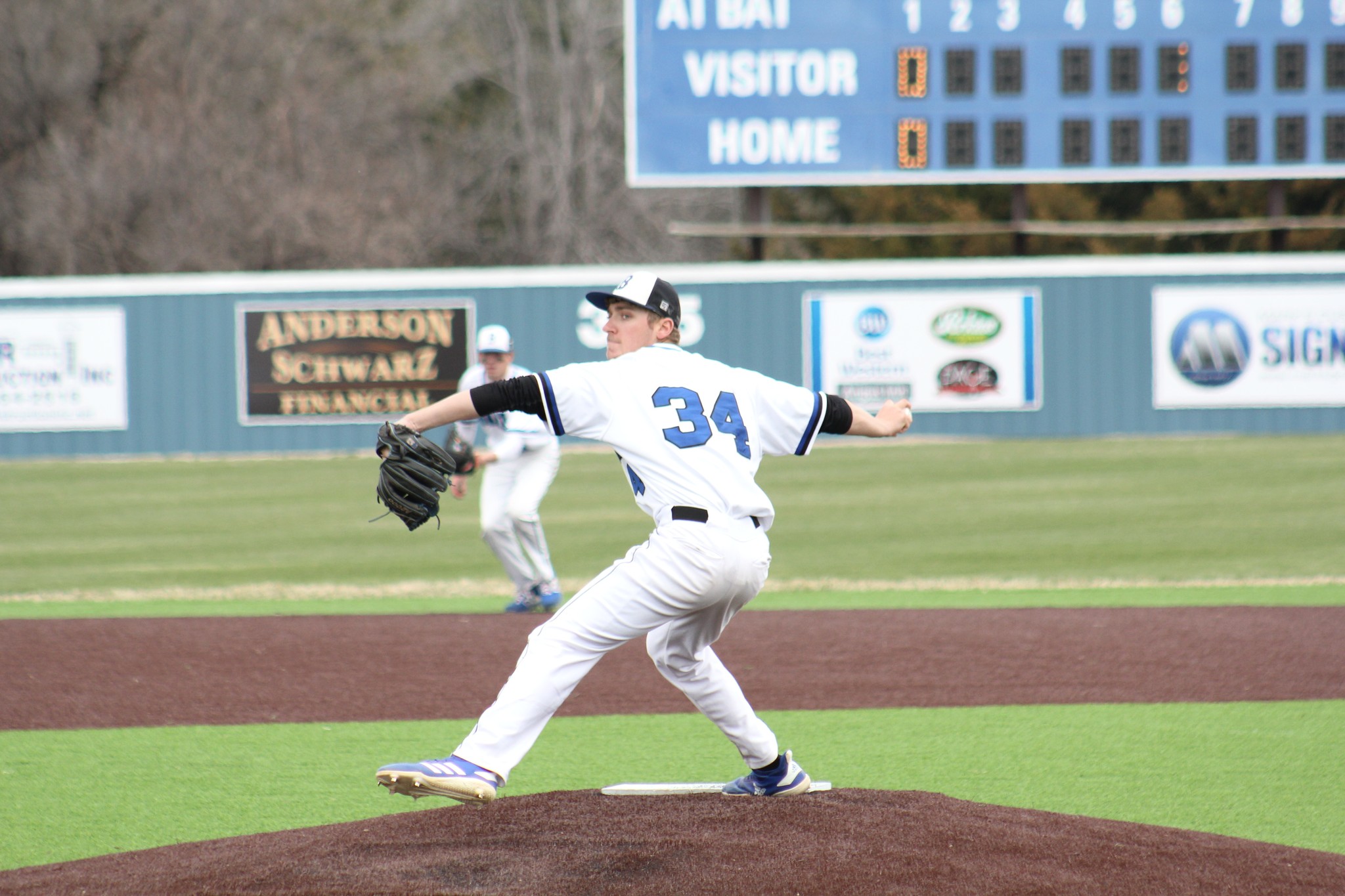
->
xmin=585 ymin=274 xmax=681 ymax=329
xmin=477 ymin=325 xmax=510 ymax=354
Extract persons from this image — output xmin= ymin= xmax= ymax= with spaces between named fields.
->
xmin=444 ymin=322 xmax=563 ymax=613
xmin=374 ymin=271 xmax=915 ymax=804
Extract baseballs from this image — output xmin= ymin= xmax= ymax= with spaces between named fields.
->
xmin=901 ymin=406 xmax=913 ymax=423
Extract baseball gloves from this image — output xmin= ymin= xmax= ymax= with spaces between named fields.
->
xmin=444 ymin=428 xmax=479 ymax=475
xmin=373 ymin=422 xmax=456 ymax=533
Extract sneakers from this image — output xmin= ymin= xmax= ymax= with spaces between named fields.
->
xmin=374 ymin=755 xmax=498 ymax=812
xmin=721 ymin=749 xmax=811 ymax=798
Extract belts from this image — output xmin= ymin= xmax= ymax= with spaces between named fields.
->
xmin=671 ymin=506 xmax=762 ymax=530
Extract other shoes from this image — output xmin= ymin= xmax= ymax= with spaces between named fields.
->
xmin=534 ymin=583 xmax=561 ymax=613
xmin=505 ymin=585 xmax=539 ymax=614
